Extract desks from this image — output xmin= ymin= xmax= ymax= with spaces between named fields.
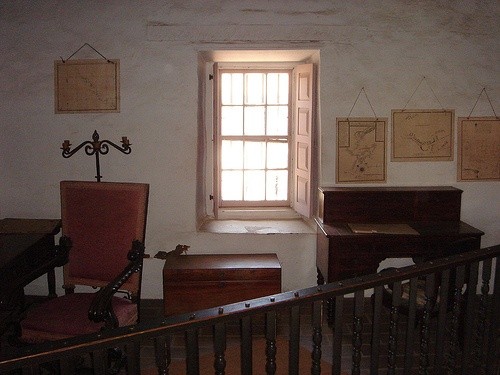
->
xmin=0 ymin=218 xmax=62 ymax=335
xmin=314 ymin=217 xmax=485 ymax=329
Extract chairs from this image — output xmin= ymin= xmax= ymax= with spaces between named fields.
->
xmin=0 ymin=180 xmax=150 ymax=375
xmin=369 ymin=238 xmax=478 ymax=360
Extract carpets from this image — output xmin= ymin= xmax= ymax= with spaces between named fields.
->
xmin=140 ymin=333 xmax=351 ymax=375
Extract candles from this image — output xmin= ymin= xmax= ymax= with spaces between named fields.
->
xmin=124 ymin=140 xmax=129 ymax=144
xmin=122 ymin=137 xmax=127 ymax=141
xmin=94 ymin=142 xmax=99 ymax=149
xmin=64 ymin=140 xmax=69 ymax=148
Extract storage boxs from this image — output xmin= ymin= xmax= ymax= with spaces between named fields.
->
xmin=163 ymin=253 xmax=281 ymax=334
